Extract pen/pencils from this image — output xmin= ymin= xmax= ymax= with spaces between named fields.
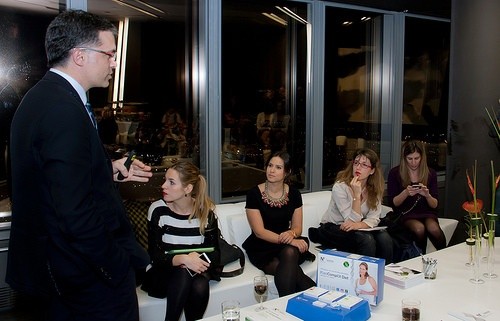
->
xmin=422 ymin=257 xmax=438 ymax=277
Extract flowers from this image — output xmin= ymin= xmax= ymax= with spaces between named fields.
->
xmin=461 ymin=158 xmax=500 ymax=263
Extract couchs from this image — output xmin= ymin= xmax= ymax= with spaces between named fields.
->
xmin=136 ymin=191 xmax=460 ymax=320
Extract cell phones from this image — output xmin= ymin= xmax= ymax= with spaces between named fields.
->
xmin=185 ymin=253 xmax=212 ymax=278
xmin=412 ymin=185 xmax=421 ymax=190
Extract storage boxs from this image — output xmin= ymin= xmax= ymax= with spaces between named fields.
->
xmin=316 ymin=247 xmax=386 ymax=307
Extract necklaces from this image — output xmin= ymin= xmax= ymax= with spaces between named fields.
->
xmin=262 ymin=179 xmax=289 ymax=207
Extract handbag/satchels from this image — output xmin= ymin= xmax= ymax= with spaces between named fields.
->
xmin=308 ymin=223 xmax=323 ymax=243
xmin=210 ymin=238 xmax=245 ymax=281
xmin=378 ymin=211 xmax=403 ymax=233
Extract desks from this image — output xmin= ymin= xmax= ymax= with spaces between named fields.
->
xmin=200 ymin=237 xmax=500 ymax=320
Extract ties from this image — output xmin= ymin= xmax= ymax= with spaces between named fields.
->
xmin=85 ymin=103 xmax=97 ymax=134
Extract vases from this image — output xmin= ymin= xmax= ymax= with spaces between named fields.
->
xmin=464 ymin=211 xmax=498 ymax=285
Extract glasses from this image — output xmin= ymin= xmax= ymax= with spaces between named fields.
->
xmin=352 ymin=160 xmax=373 ymax=169
xmin=69 ymin=46 xmax=117 ymax=61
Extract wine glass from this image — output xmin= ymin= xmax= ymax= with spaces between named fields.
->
xmin=252 ymin=276 xmax=269 ymax=310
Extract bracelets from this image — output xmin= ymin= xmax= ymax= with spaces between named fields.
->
xmin=352 ymin=195 xmax=360 ymax=200
xmin=289 ymin=230 xmax=298 ymax=238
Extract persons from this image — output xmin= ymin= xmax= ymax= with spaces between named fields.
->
xmin=3 ymin=6 xmax=154 ymax=321
xmin=354 ymin=261 xmax=377 ymax=305
xmin=386 ymin=139 xmax=448 ymax=256
xmin=316 ymin=146 xmax=394 ymax=270
xmin=145 ymin=160 xmax=225 ymax=320
xmin=94 ymin=83 xmax=294 ymax=161
xmin=240 ymin=150 xmax=318 ymax=298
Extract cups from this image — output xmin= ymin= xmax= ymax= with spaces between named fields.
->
xmin=221 ymin=301 xmax=240 ymax=321
xmin=402 ymin=301 xmax=420 ymax=321
xmin=424 ymin=255 xmax=437 ymax=279
